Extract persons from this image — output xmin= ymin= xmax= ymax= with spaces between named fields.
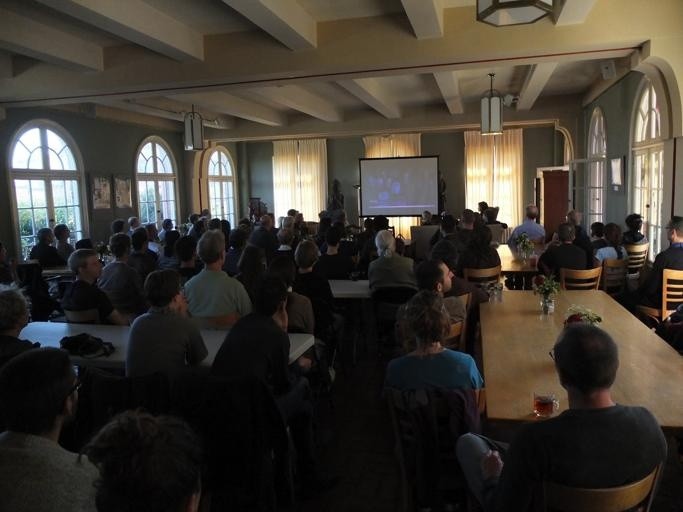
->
xmin=622 ymin=214 xmax=647 ymax=274
xmin=266 ymin=272 xmax=315 ymax=333
xmin=95 ymin=233 xmax=145 ymax=317
xmin=507 ymin=204 xmax=546 ymax=288
xmin=399 ymin=257 xmax=467 ymax=350
xmin=459 ymin=210 xmax=482 ymax=283
xmin=0 ymin=345 xmax=110 ymax=511
xmin=129 ymin=227 xmax=158 ymax=281
xmin=565 ymin=209 xmax=589 ymax=242
xmin=160 ymin=234 xmax=202 ymax=285
xmin=54 ymin=222 xmax=74 ymax=258
xmin=126 ymin=269 xmax=209 ymax=394
xmin=145 ymin=223 xmax=164 ymax=256
xmin=473 ymin=211 xmax=492 ymax=246
xmin=212 ymin=281 xmax=313 ymax=420
xmin=76 ymin=239 xmax=92 ymax=251
xmin=369 ymin=229 xmax=419 ymax=336
xmin=318 ymin=230 xmax=350 ymax=281
xmin=356 ymin=216 xmax=389 ymax=270
xmin=282 ymin=216 xmax=298 ymax=235
xmin=432 ymin=214 xmax=465 ymax=256
xmin=268 ymin=229 xmax=295 ymax=261
xmin=621 ymin=216 xmax=683 ymax=311
xmin=95 ymin=415 xmax=202 ymax=512
xmin=421 ymin=210 xmax=434 ymax=225
xmin=288 ymin=209 xmax=307 ymax=240
xmin=239 ymin=219 xmax=253 ymax=240
xmin=108 ymin=219 xmax=126 ymax=250
xmin=293 ymin=239 xmax=340 ymax=384
xmin=431 ymin=238 xmax=489 ymax=304
xmin=482 ymin=207 xmax=500 ymax=226
xmin=224 ymin=223 xmax=250 ymax=276
xmin=159 ymin=219 xmax=173 ymax=242
xmin=539 ymin=222 xmax=587 ymax=289
xmin=595 ymin=223 xmax=628 ymax=291
xmin=27 ymin=227 xmax=59 ymax=269
xmin=250 ymin=214 xmax=277 ymax=259
xmin=382 ymin=289 xmax=484 ymax=407
xmin=60 ymin=248 xmax=127 ymax=326
xmin=128 ymin=216 xmax=141 ymax=227
xmin=0 ymin=281 xmax=41 ymax=361
xmin=589 ymin=222 xmax=606 ymax=248
xmin=188 ymin=209 xmax=231 ymax=239
xmin=182 ymin=231 xmax=253 ymax=328
xmin=457 ymin=321 xmax=668 ymax=508
xmin=478 ymin=202 xmax=489 ymax=215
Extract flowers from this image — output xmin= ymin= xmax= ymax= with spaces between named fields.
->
xmin=563 ymin=304 xmax=602 ymax=328
xmin=534 ymin=274 xmax=561 ymax=300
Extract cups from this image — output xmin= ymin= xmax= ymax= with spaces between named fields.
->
xmin=533 ymin=391 xmax=559 ymax=418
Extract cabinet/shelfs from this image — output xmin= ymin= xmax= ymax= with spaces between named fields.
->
xmin=533 ymin=169 xmax=569 ymax=240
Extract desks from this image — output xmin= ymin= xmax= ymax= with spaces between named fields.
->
xmin=496 ymin=244 xmax=544 ymax=271
xmin=340 ymin=238 xmax=415 ymax=247
xmin=17 ymin=322 xmax=315 ymax=367
xmin=328 ymin=280 xmax=372 ymax=299
xmin=411 ymin=224 xmax=462 ymax=258
xmin=479 ymin=290 xmax=683 ymax=426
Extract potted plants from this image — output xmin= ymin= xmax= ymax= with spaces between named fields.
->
xmin=516 ymin=232 xmax=537 ymax=265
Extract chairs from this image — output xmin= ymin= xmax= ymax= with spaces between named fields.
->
xmin=635 ymin=268 xmax=682 ymax=324
xmin=457 ymin=292 xmax=470 ymax=312
xmin=559 ymin=267 xmax=601 ymax=291
xmin=602 ymin=259 xmax=624 ymax=291
xmin=443 ymin=322 xmax=464 ymax=350
xmin=463 ymin=265 xmax=502 ymax=293
xmin=626 ymin=242 xmax=649 ymax=270
xmin=372 ymin=286 xmax=420 ymax=362
xmin=346 ymin=224 xmax=362 ymax=238
xmin=383 ymin=386 xmax=485 ymax=512
xmin=541 ymin=462 xmax=661 ymax=512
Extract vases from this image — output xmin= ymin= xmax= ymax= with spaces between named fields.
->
xmin=541 ymin=300 xmax=554 ymax=313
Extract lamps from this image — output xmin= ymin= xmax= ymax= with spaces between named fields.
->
xmin=475 ymin=1 xmax=552 ymax=27
xmin=183 ymin=104 xmax=204 ymax=151
xmin=480 ymin=72 xmax=504 ymax=135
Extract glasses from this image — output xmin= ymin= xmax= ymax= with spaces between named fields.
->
xmin=68 ymin=381 xmax=79 ymax=398
xmin=549 ymin=348 xmax=557 ymax=360
